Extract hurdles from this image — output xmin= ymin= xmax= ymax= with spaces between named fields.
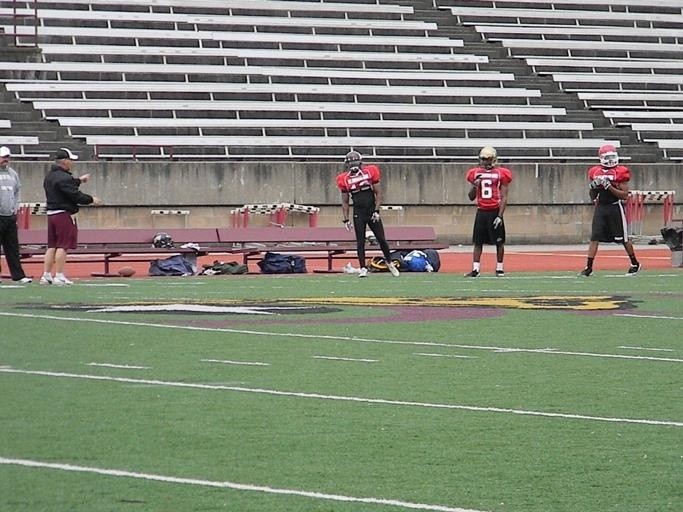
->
xmin=230 ymin=203 xmax=319 ymax=229
xmin=16 ymin=202 xmax=49 ymax=230
xmin=150 ymin=209 xmax=191 ymax=228
xmin=624 ymin=189 xmax=676 ymax=244
xmin=380 ymin=206 xmax=403 ymax=224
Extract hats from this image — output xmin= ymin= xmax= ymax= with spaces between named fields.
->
xmin=0 ymin=146 xmax=11 ymax=157
xmin=54 ymin=148 xmax=78 ymax=160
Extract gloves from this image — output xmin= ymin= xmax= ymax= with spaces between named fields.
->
xmin=371 ymin=212 xmax=381 ymax=224
xmin=493 ymin=217 xmax=502 ymax=229
xmin=589 ymin=176 xmax=611 ymax=190
xmin=345 ymin=221 xmax=354 ymax=232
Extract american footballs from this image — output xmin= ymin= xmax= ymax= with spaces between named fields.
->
xmin=118 ymin=268 xmax=134 ymax=277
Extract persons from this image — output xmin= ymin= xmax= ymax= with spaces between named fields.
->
xmin=578 ymin=144 xmax=641 ymax=276
xmin=0 ymin=147 xmax=33 ymax=286
xmin=464 ymin=146 xmax=513 ymax=278
xmin=39 ymin=147 xmax=103 ymax=286
xmin=337 ymin=151 xmax=401 ymax=278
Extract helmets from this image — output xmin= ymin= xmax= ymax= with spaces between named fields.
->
xmin=479 ymin=146 xmax=498 ymax=169
xmin=345 ymin=151 xmax=362 ymax=172
xmin=599 ymin=145 xmax=619 ymax=168
xmin=153 ymin=232 xmax=173 ymax=248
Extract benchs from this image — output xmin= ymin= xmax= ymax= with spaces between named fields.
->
xmin=217 ymin=225 xmax=450 ymax=273
xmin=0 ymin=2 xmax=683 ymax=166
xmin=0 ymin=228 xmax=217 ymax=278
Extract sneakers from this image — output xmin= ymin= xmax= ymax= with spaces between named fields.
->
xmin=385 ymin=260 xmax=400 ymax=277
xmin=358 ymin=267 xmax=368 ymax=277
xmin=464 ymin=263 xmax=641 ymax=277
xmin=12 ymin=276 xmax=73 ymax=286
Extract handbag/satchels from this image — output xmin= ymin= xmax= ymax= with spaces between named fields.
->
xmin=149 ymin=255 xmax=193 ymax=276
xmin=368 ymin=256 xmax=400 ymax=271
xmin=257 ymin=252 xmax=308 ymax=274
